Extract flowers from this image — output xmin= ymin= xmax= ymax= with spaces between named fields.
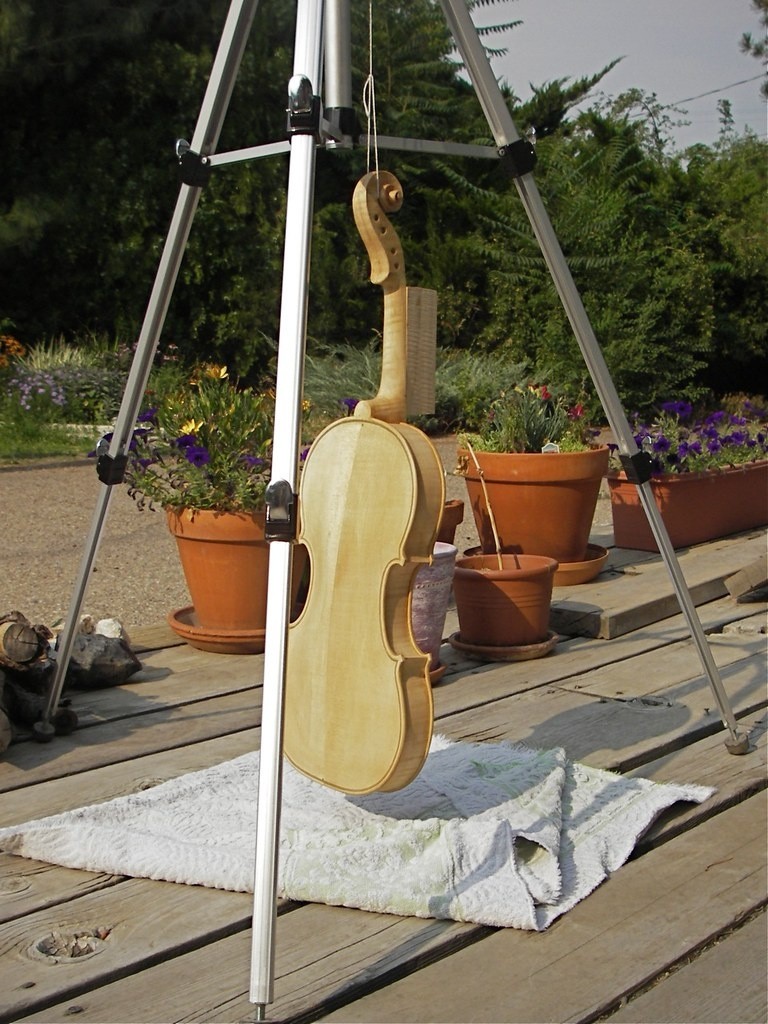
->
xmin=456 ymin=382 xmax=603 ymax=454
xmin=588 ymin=399 xmax=768 ymax=477
xmin=92 ymin=352 xmax=315 ymax=523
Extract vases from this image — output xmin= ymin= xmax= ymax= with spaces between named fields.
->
xmin=163 ymin=502 xmax=309 ymax=629
xmin=604 ymin=458 xmax=768 ymax=553
xmin=440 ymin=501 xmax=466 ymax=544
xmin=455 ymin=443 xmax=611 ymax=560
xmin=409 ymin=542 xmax=458 ymax=670
xmin=451 ymin=553 xmax=560 ymax=646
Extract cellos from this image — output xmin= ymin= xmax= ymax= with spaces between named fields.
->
xmin=275 ymin=164 xmax=447 ymax=800
